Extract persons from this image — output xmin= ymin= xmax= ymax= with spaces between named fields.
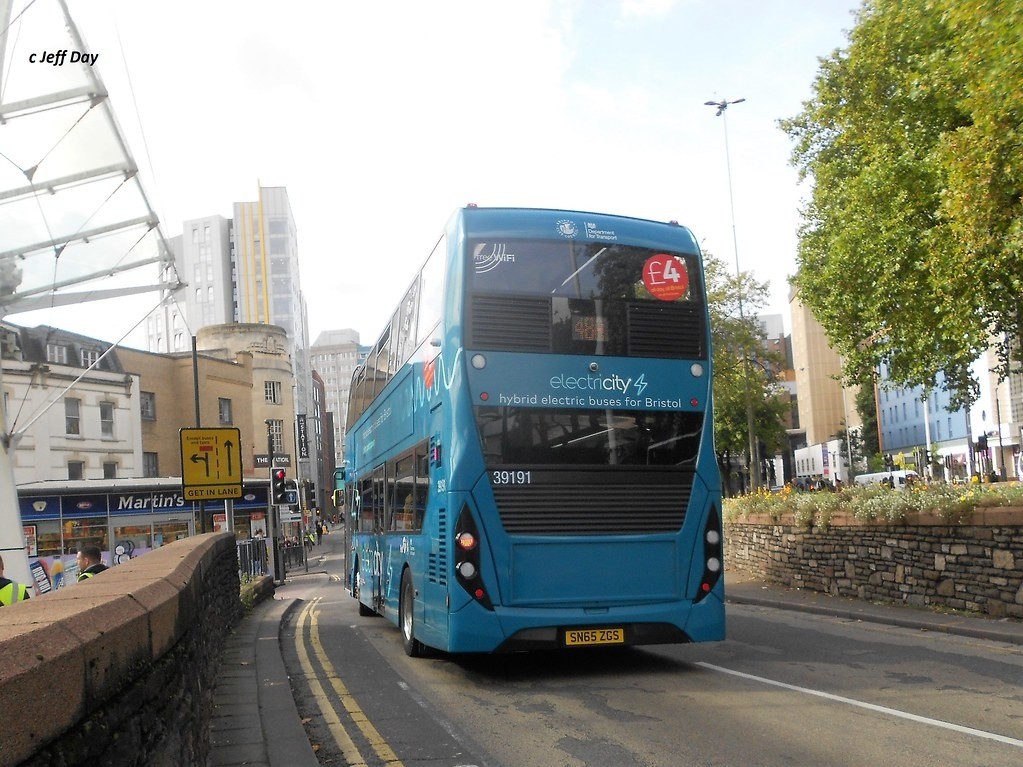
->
xmin=310 ymin=527 xmax=315 ymax=537
xmin=284 ymin=535 xmax=291 ymax=569
xmin=881 ymin=477 xmax=888 ymax=484
xmin=316 ymin=524 xmax=323 ymax=546
xmin=254 ymin=529 xmax=263 ymax=540
xmin=75 ymin=546 xmax=109 ymax=581
xmin=0 ymin=555 xmax=31 ymax=607
xmin=889 ymin=475 xmax=896 ymax=489
xmin=814 ymin=474 xmax=826 ymax=491
xmin=791 ymin=477 xmax=805 ymax=493
xmin=292 ymin=535 xmax=302 ymax=566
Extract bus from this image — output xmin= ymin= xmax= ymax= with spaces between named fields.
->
xmin=342 ymin=202 xmax=728 ymax=660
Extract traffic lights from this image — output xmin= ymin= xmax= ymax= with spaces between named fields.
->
xmin=978 ymin=435 xmax=986 ymax=450
xmin=976 ymin=441 xmax=981 ymax=452
xmin=269 ymin=466 xmax=286 ymax=505
xmin=926 ymin=449 xmax=931 ymax=465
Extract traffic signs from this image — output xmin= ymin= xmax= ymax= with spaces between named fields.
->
xmin=178 ymin=427 xmax=243 ymax=501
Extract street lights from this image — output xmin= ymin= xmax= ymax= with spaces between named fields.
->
xmin=705 ymin=96 xmax=762 ymax=494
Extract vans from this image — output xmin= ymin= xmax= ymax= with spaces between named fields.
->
xmin=853 ymin=469 xmax=921 ymax=491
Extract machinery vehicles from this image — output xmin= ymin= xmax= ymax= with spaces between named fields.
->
xmin=15 ymin=475 xmax=283 ymax=584
